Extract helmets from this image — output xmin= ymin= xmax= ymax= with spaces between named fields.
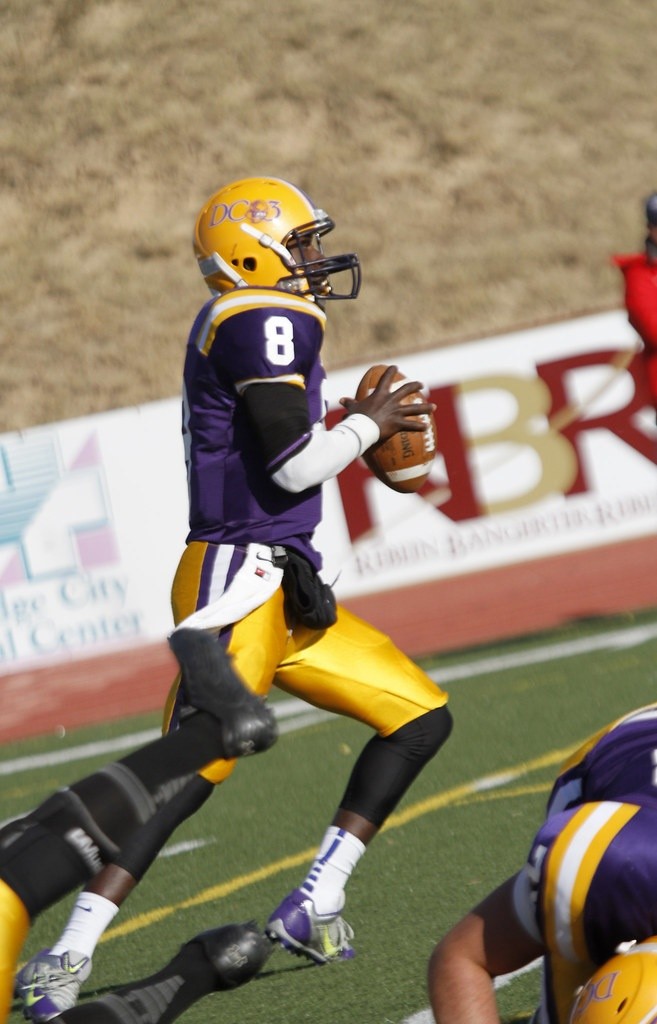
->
xmin=190 ymin=176 xmax=331 ymax=306
xmin=571 ymin=938 xmax=657 ymax=1022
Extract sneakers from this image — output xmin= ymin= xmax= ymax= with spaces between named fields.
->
xmin=264 ymin=887 xmax=355 ymax=964
xmin=12 ymin=947 xmax=93 ymax=1024
xmin=170 ymin=626 xmax=279 ymax=761
xmin=188 ymin=918 xmax=272 ymax=990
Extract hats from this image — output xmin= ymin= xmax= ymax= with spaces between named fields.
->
xmin=646 ymin=191 xmax=657 ymax=226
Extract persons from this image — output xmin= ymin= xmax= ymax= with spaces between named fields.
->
xmin=0 ymin=176 xmax=453 ymax=964
xmin=0 ymin=628 xmax=279 ymax=1024
xmin=611 ymin=192 xmax=657 ymax=427
xmin=427 ymin=702 xmax=657 ymax=1024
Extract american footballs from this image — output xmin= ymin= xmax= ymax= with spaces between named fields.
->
xmin=354 ymin=363 xmax=439 ymax=494
